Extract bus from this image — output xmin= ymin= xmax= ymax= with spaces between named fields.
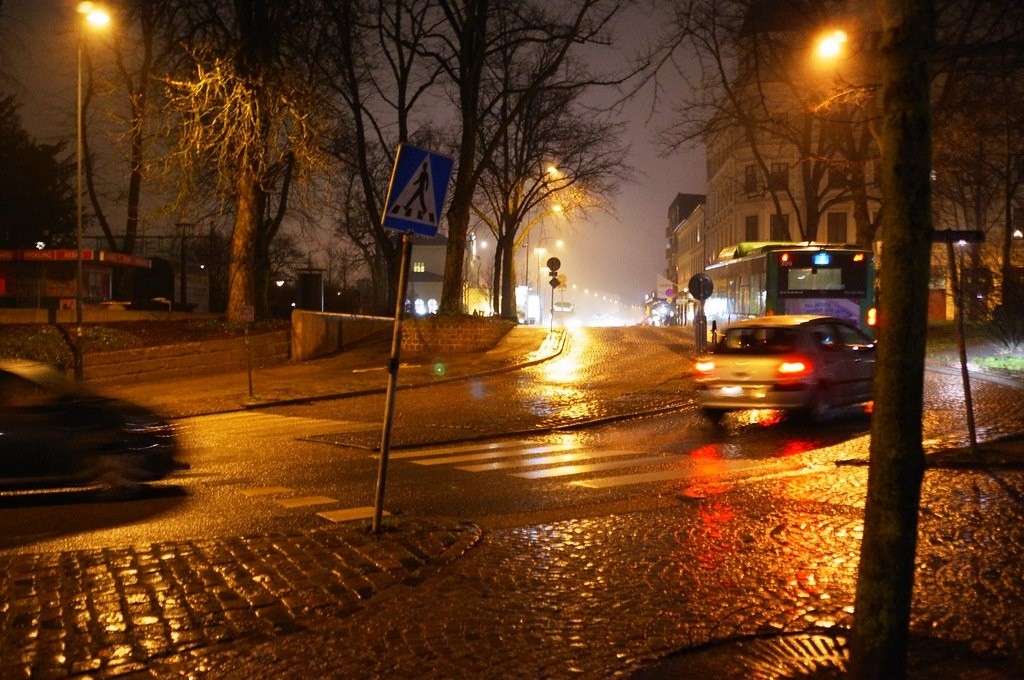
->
xmin=703 ymin=240 xmax=877 ymax=340
xmin=98 ymin=296 xmax=171 ymax=311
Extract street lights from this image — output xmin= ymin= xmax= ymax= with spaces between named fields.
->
xmin=810 ymin=27 xmax=883 ymax=255
xmin=72 ymin=0 xmax=121 ymax=376
xmin=522 ymin=167 xmax=567 ymax=324
xmin=34 ymin=237 xmax=46 ymax=310
xmin=175 ymin=219 xmax=194 ymax=311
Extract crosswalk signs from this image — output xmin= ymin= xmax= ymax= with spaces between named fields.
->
xmin=380 ymin=141 xmax=456 ymax=240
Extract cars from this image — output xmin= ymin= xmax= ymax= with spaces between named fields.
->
xmin=691 ymin=313 xmax=881 ymax=423
xmin=0 ymin=352 xmax=182 ymax=506
